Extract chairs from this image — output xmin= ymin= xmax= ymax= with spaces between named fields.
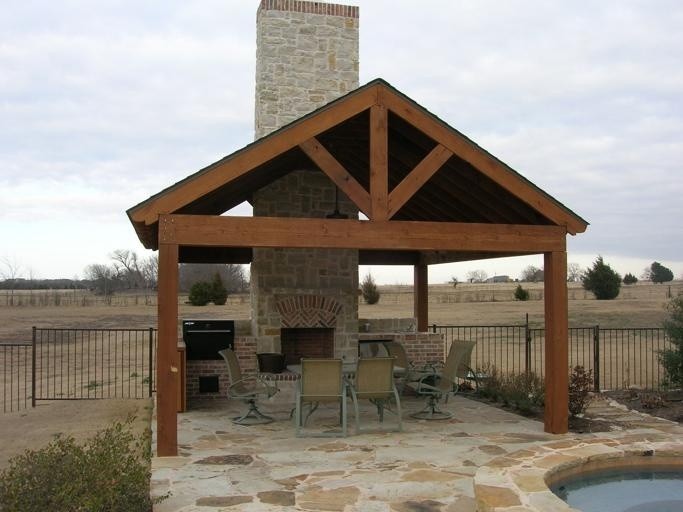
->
xmin=432 ymin=341 xmax=478 ymax=397
xmin=383 ymin=342 xmax=405 ymax=366
xmin=406 ymin=336 xmax=476 ymax=420
xmin=344 ymin=356 xmax=404 ymax=435
xmin=295 ymin=357 xmax=347 ymax=437
xmin=218 ymin=348 xmax=279 ymax=426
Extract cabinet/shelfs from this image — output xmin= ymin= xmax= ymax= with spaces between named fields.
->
xmin=176 ymin=339 xmax=186 ymax=414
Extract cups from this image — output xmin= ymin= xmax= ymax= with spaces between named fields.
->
xmin=364 ymin=323 xmax=370 ymax=332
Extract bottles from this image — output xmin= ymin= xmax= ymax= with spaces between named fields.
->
xmin=432 ymin=324 xmax=436 ymax=333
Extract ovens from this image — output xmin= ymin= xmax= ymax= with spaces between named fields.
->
xmin=181 ymin=318 xmax=234 ymax=361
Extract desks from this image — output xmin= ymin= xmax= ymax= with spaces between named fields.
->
xmin=256 ymin=363 xmax=407 ymax=428
